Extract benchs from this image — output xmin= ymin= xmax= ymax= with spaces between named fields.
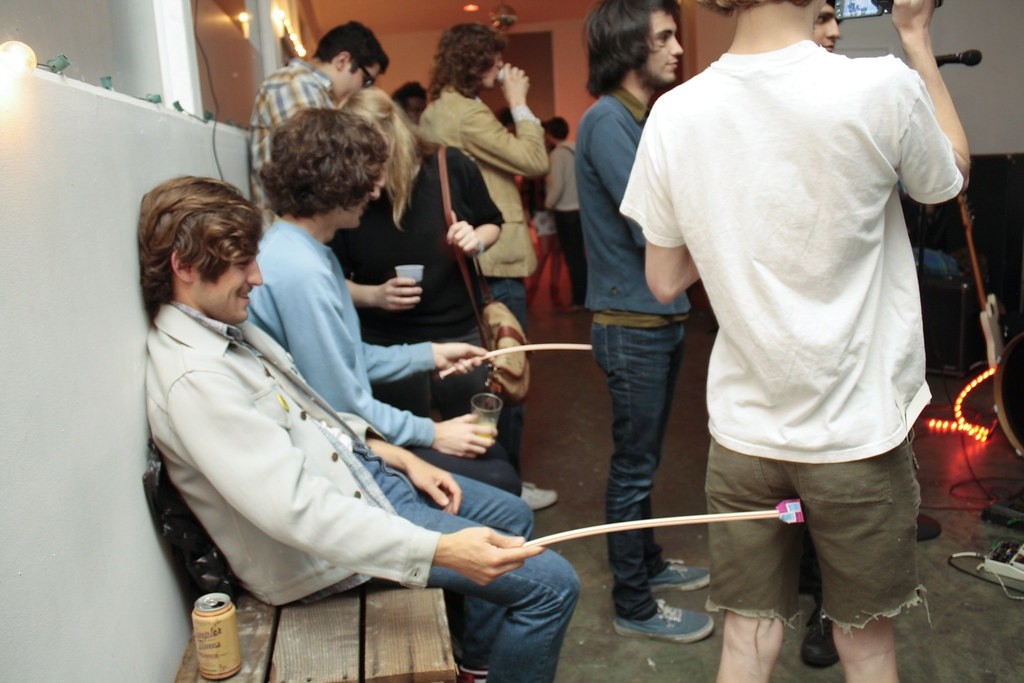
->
xmin=175 ymin=587 xmax=461 ymax=683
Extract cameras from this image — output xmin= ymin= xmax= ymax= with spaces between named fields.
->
xmin=834 ymin=0 xmax=944 ymax=21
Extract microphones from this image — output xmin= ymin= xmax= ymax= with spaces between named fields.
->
xmin=935 ymin=50 xmax=982 ymax=66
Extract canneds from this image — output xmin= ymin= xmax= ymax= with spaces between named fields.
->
xmin=191 ymin=593 xmax=242 ymax=680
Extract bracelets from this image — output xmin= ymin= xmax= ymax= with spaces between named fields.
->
xmin=472 ymin=236 xmax=484 ymax=258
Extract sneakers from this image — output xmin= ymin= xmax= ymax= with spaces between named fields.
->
xmin=647 ymin=564 xmax=712 ymax=590
xmin=613 ymin=599 xmax=713 ymax=643
xmin=802 ymin=601 xmax=839 ymax=667
xmin=914 ymin=513 xmax=942 ymax=542
xmin=519 ymin=480 xmax=557 ymax=511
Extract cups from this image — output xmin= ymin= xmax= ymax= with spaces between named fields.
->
xmin=470 ymin=392 xmax=503 ymax=438
xmin=497 ymin=67 xmax=505 ymax=85
xmin=395 ymin=265 xmax=424 ymax=305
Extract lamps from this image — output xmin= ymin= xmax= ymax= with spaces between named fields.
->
xmin=488 ymin=0 xmax=517 ymax=33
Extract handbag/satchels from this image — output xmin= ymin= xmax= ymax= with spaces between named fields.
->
xmin=476 ymin=296 xmax=529 ymax=406
xmin=142 ymin=438 xmax=241 ymax=627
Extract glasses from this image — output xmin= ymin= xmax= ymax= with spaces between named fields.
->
xmin=343 ymin=50 xmax=375 ymax=87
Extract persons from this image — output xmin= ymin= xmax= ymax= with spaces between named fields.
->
xmin=138 ymin=175 xmax=581 ymax=683
xmin=576 ymin=0 xmax=715 ymax=642
xmin=240 ymin=17 xmax=577 ymax=509
xmin=618 ymin=0 xmax=970 ymax=683
